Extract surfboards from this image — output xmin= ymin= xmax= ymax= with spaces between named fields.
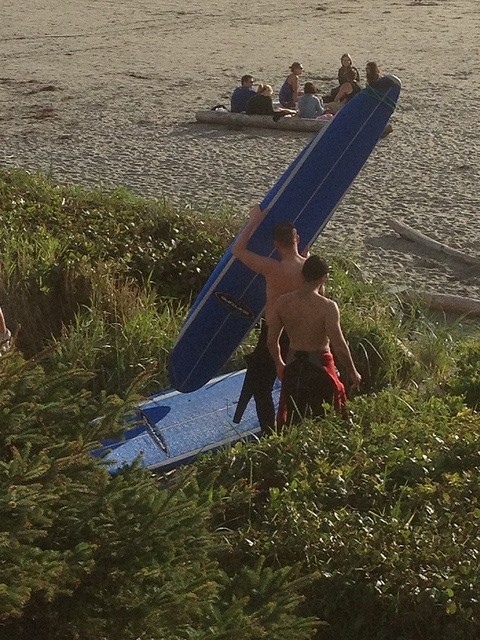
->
xmin=166 ymin=73 xmax=402 ymax=394
xmin=85 ymin=364 xmax=341 ymax=482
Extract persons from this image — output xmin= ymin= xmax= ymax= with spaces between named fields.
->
xmin=334 ymin=69 xmax=362 ymax=103
xmin=268 ymin=255 xmax=361 ymax=436
xmin=338 ymin=54 xmax=360 ymax=84
xmin=280 ymin=62 xmax=303 ymax=108
xmin=246 ymin=85 xmax=282 ymax=113
xmin=299 ymin=83 xmax=325 ymax=118
xmin=365 ymin=62 xmax=381 ymax=83
xmin=232 ymin=204 xmax=325 ymax=436
xmin=231 ymin=75 xmax=257 ymax=112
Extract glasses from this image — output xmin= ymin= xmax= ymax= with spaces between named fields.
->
xmin=250 ymin=81 xmax=254 ymax=83
xmin=301 ymin=68 xmax=303 ymax=69
